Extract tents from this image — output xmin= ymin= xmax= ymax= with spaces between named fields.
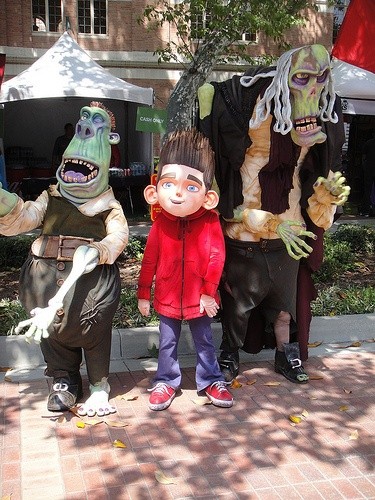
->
xmin=330 ymin=55 xmax=375 ymax=220
xmin=0 ymin=31 xmax=154 ymax=219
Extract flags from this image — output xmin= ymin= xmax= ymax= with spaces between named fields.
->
xmin=331 ymin=0 xmax=375 ymax=73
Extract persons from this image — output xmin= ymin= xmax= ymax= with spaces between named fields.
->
xmin=138 ymin=131 xmax=234 ymax=410
xmin=196 ymin=45 xmax=351 ymax=385
xmin=0 ymin=100 xmax=129 ymax=416
